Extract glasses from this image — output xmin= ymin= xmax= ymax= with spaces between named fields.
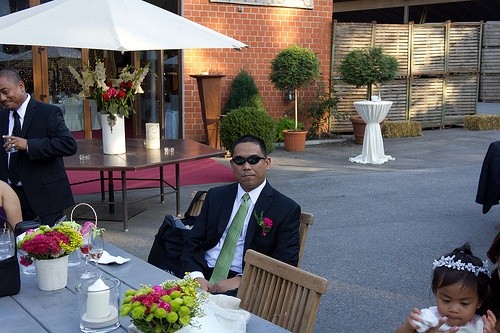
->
xmin=232 ymin=156 xmax=267 ymax=165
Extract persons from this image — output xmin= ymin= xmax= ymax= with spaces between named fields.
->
xmin=0 ymin=70 xmax=77 ymax=228
xmin=0 ymin=180 xmax=23 ymax=231
xmin=177 ymin=133 xmax=301 ymax=297
xmin=395 ymin=232 xmax=500 ymax=333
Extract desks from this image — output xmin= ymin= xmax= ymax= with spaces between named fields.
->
xmin=62 ymin=138 xmax=228 ymax=232
xmin=0 ymin=235 xmax=291 ymax=333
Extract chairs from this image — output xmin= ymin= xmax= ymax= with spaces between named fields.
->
xmin=182 ymin=189 xmax=207 ymax=217
xmin=294 ymin=212 xmax=314 ymax=267
xmin=235 ymin=248 xmax=328 ymax=333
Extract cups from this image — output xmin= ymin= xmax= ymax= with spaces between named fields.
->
xmin=4 ymin=134 xmax=18 ymax=153
xmin=74 ymin=277 xmax=122 ymax=333
xmin=0 ymin=227 xmax=40 ymax=274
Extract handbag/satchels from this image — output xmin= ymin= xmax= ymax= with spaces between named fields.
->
xmin=0 ymin=215 xmax=21 ymax=298
xmin=147 ymin=215 xmax=196 ymax=278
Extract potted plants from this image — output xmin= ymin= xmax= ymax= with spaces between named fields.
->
xmin=269 ymin=45 xmax=321 ymax=153
xmin=340 ymin=47 xmax=398 ymax=145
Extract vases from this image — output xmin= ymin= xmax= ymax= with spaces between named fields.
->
xmin=33 ymin=257 xmax=68 ymax=291
xmin=100 ymin=113 xmax=126 ymax=155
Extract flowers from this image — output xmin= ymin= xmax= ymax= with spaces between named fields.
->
xmin=120 ymin=274 xmax=203 ymax=333
xmin=16 ymin=221 xmax=82 ymax=266
xmin=67 ymin=58 xmax=149 ymax=123
xmin=254 ymin=213 xmax=272 ymax=237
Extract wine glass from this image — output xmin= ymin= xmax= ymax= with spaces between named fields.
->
xmin=79 ymin=224 xmax=104 ymax=282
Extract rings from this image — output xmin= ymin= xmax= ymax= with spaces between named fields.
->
xmin=13 ymin=144 xmax=14 ymax=147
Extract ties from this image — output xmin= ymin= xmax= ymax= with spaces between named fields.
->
xmin=8 ymin=111 xmax=21 ymax=185
xmin=209 ymin=194 xmax=250 ymax=285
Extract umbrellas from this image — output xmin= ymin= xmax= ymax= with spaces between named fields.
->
xmin=9 ymin=47 xmax=82 ymax=94
xmin=0 ymin=0 xmax=249 ymax=139
